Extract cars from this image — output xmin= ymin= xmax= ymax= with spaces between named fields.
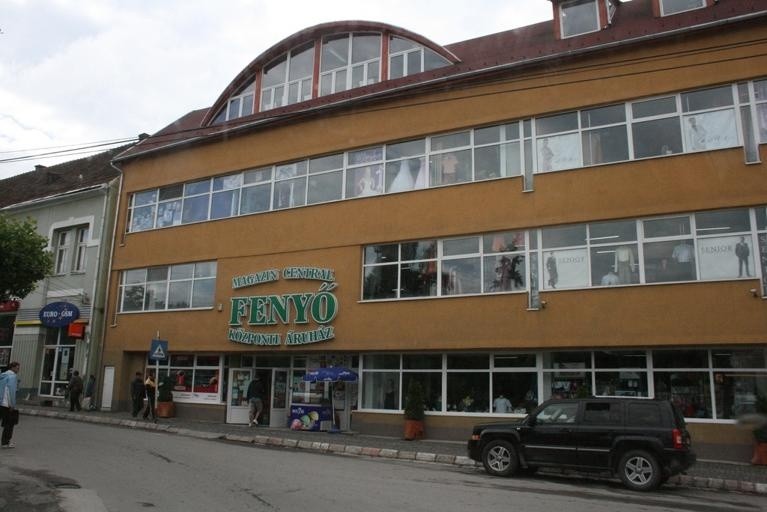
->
xmin=466 ymin=397 xmax=697 ymax=491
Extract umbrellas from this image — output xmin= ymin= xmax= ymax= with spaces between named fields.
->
xmin=304 ymin=365 xmax=358 ymax=425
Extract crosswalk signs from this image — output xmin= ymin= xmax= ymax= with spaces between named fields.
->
xmin=150 ymin=339 xmax=168 ymax=360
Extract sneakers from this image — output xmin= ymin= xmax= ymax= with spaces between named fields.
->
xmin=249 ymin=423 xmax=255 ymax=427
xmin=2 ymin=443 xmax=15 ymax=448
xmin=253 ymin=419 xmax=258 ymax=425
xmin=133 ymin=415 xmax=159 ymax=423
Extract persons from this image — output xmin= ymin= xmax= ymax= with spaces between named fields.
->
xmin=687 ymin=117 xmax=709 ymax=151
xmin=546 ymin=252 xmax=559 ymax=290
xmin=140 ymin=376 xmax=159 ymax=423
xmin=0 ymin=362 xmax=21 ymax=448
xmin=67 ymin=370 xmax=82 ymax=411
xmin=736 ymin=237 xmax=753 ymax=278
xmin=247 ymin=378 xmax=266 ymax=429
xmin=539 ymin=138 xmax=557 ymax=170
xmin=358 ymin=166 xmax=379 ymax=196
xmin=614 ymin=244 xmax=636 ymax=283
xmin=493 ymin=394 xmax=512 ymax=412
xmin=599 ymin=271 xmax=619 ymax=285
xmin=673 ymin=240 xmax=694 ymax=279
xmin=130 ymin=371 xmax=145 ymax=419
